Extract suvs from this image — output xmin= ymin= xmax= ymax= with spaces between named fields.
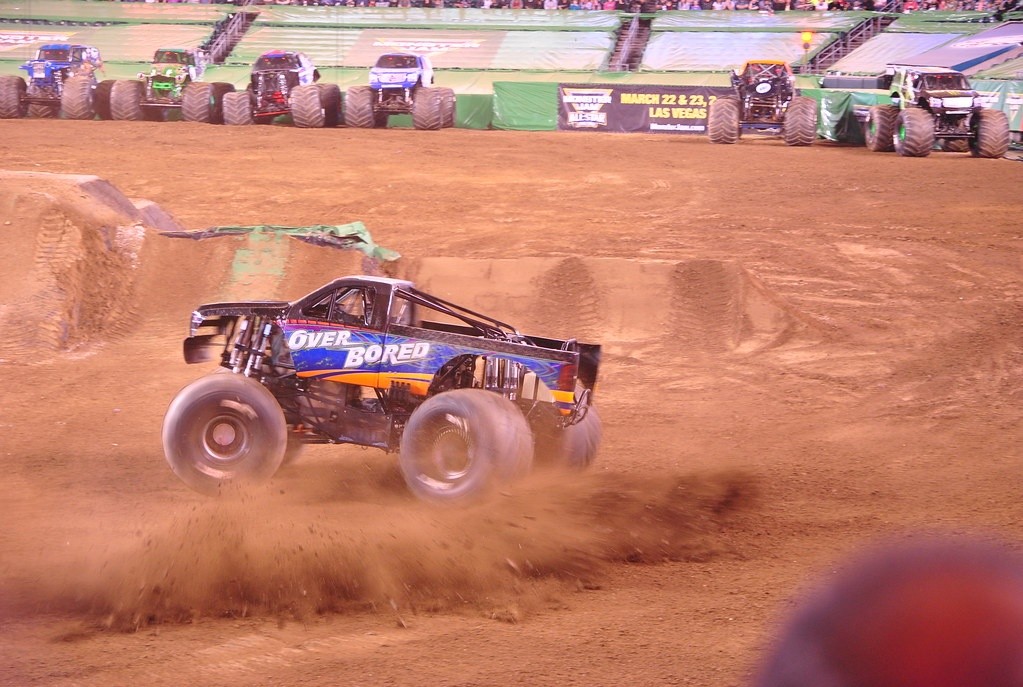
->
xmin=162 ymin=274 xmax=603 ymax=500
xmin=110 ymin=47 xmax=236 ymax=124
xmin=345 ymin=52 xmax=456 ymax=128
xmin=708 ymin=59 xmax=817 ymax=146
xmin=0 ymin=44 xmax=117 ymax=120
xmin=223 ymin=50 xmax=341 ymax=128
xmin=863 ymin=65 xmax=1009 ymax=158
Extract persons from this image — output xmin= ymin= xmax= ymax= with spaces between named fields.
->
xmin=655 ymin=0 xmax=901 ymax=17
xmin=111 ymin=0 xmax=647 ymax=13
xmin=334 ymin=287 xmax=378 ymax=325
xmin=902 ymin=0 xmax=1023 ymax=22
